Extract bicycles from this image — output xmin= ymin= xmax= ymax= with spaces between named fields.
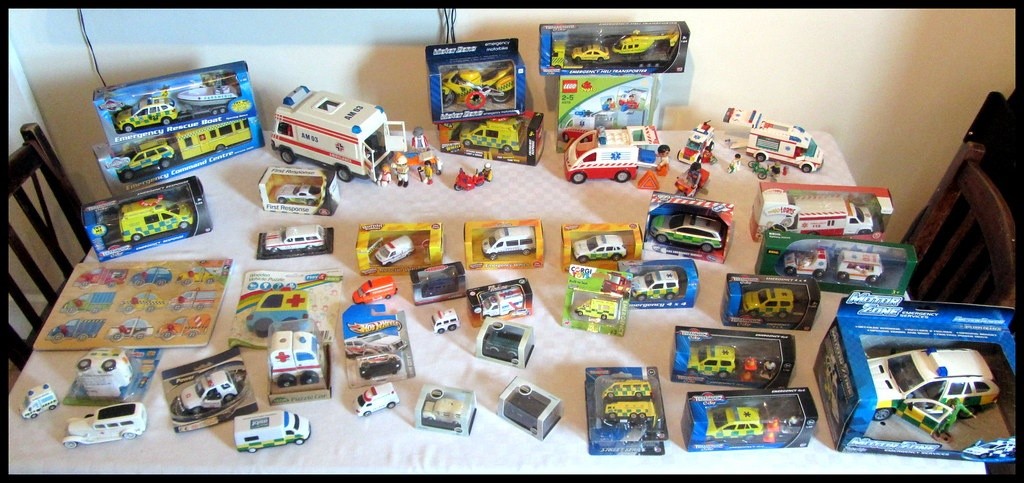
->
xmin=748 ymin=160 xmax=767 ymax=179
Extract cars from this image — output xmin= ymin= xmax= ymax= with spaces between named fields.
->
xmin=360 ymin=353 xmax=402 ymax=380
xmin=572 ymin=45 xmax=610 ymax=62
xmin=574 ymin=234 xmax=626 ymax=263
xmin=116 ymin=99 xmax=177 ymax=132
xmin=276 ymin=184 xmax=321 ymax=206
xmin=62 ymin=402 xmax=148 ymax=449
xmin=676 ymin=121 xmax=716 ymax=164
xmin=345 ymin=331 xmax=408 ymax=358
xmin=784 ymin=247 xmax=886 ymax=285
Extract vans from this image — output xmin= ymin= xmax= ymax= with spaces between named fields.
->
xmin=234 ymin=410 xmax=311 ymax=453
xmin=431 ymin=307 xmax=460 ymax=333
xmin=120 ymin=199 xmax=195 ymax=242
xmin=481 ymin=227 xmax=535 ymax=260
xmin=353 ymin=381 xmax=400 ymax=417
xmin=601 ymin=380 xmax=653 ymax=399
xmin=375 ymin=235 xmax=414 ymax=266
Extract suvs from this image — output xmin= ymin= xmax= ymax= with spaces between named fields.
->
xmin=632 ymin=270 xmax=680 ymax=301
xmin=742 ymin=288 xmax=794 ymax=318
xmin=117 ymin=145 xmax=175 ymax=179
xmin=649 ymin=212 xmax=725 ymax=253
xmin=867 ymin=347 xmax=1001 ymax=436
xmin=689 ymin=346 xmax=736 ymax=380
xmin=708 ymin=407 xmax=764 ymax=439
xmin=265 ymin=226 xmax=326 ymax=254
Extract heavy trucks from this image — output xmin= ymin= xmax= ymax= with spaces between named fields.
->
xmin=600 ymin=271 xmax=631 ymax=300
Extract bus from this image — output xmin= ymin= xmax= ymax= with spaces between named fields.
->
xmin=175 ymin=117 xmax=252 ymax=161
xmin=422 ymin=278 xmax=459 ymax=298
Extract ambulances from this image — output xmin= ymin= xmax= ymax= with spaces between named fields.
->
xmin=271 ymin=85 xmax=407 ymax=182
xmin=268 ymin=331 xmax=324 ymax=388
xmin=176 ymin=371 xmax=238 ymax=414
xmin=723 ymin=107 xmax=824 ymax=172
xmin=564 ymin=126 xmax=659 ymax=185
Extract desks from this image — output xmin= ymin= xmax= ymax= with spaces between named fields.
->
xmin=9 ymin=127 xmax=990 ymax=476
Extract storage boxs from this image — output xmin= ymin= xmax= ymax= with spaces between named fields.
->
xmin=539 ymin=21 xmax=690 ymax=155
xmin=560 ymin=222 xmax=700 ymax=339
xmin=415 ymin=384 xmax=478 ymax=437
xmin=680 ymin=386 xmax=819 ymax=453
xmin=755 ymin=230 xmax=918 ymax=297
xmin=719 ymin=272 xmax=821 ymax=332
xmin=669 ymin=325 xmax=797 ymax=390
xmin=497 ymin=376 xmax=564 ymax=442
xmin=91 ymin=60 xmax=267 ymax=198
xmin=424 ymin=38 xmax=546 ymax=167
xmin=257 ymin=165 xmax=342 ymax=216
xmin=475 ymin=315 xmax=535 ymax=369
xmin=79 ymin=176 xmax=215 ymax=263
xmin=812 ymin=289 xmax=1015 ymax=464
xmin=641 ymin=191 xmax=736 ymax=265
xmin=466 ymin=278 xmax=533 ymax=328
xmin=749 ymin=182 xmax=894 ymax=244
xmin=354 ymin=222 xmax=445 ymax=277
xmin=462 ymin=219 xmax=545 ymax=270
xmin=409 ymin=261 xmax=467 ymax=306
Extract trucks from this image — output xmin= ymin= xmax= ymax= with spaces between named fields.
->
xmin=793 ymin=195 xmax=884 ymax=239
xmin=423 ymin=399 xmax=463 ymax=426
xmin=460 ymin=118 xmax=525 ymax=151
xmin=352 ymin=276 xmax=396 ymax=304
xmin=575 ymin=298 xmax=618 ymax=320
xmin=482 ymin=287 xmax=524 ymax=319
xmin=20 ymin=383 xmax=58 ymax=418
xmin=605 ymin=401 xmax=656 ymax=425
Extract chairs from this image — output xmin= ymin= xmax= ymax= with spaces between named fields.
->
xmin=8 ymin=122 xmax=94 ymax=373
xmin=902 ymin=140 xmax=1017 ymax=307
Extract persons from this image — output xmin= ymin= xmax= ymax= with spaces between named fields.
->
xmin=654 ymin=142 xmax=783 ymax=193
xmin=480 ymin=163 xmax=494 ymax=182
xmin=377 ymin=124 xmax=443 ymax=188
xmin=935 ymin=398 xmax=976 ymax=438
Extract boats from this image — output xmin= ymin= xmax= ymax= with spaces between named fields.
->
xmin=177 ymin=84 xmax=238 ymax=106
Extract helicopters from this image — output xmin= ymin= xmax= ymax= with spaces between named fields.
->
xmin=612 ymin=30 xmax=679 ymax=55
